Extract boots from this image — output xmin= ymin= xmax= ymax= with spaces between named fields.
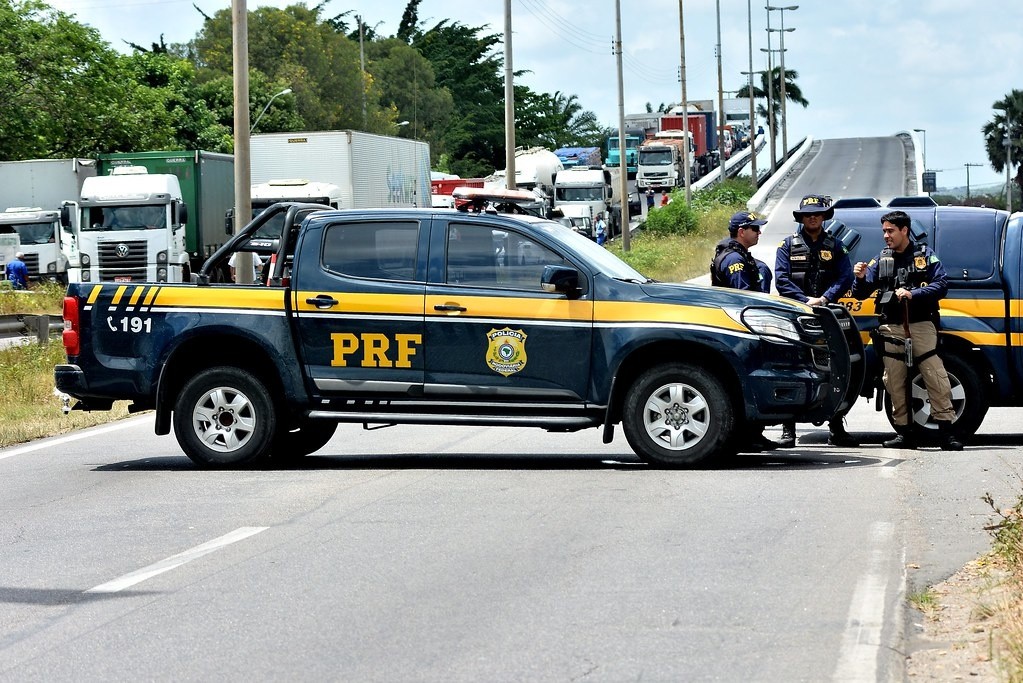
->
xmin=938 ymin=420 xmax=963 ymax=451
xmin=780 ymin=422 xmax=797 ymax=447
xmin=882 ymin=423 xmax=914 ymax=448
xmin=827 ymin=417 xmax=860 ymax=448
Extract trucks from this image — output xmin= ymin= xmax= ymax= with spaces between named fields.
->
xmin=0 ymin=158 xmax=97 ymax=286
xmin=56 ymin=148 xmax=236 ymax=286
xmin=432 ymin=145 xmax=642 ymax=246
xmin=225 ymin=130 xmax=434 ymax=278
xmin=604 ymin=97 xmax=762 ymax=194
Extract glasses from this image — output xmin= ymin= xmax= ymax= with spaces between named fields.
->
xmin=802 ymin=211 xmax=822 ymax=218
xmin=746 ymin=227 xmax=760 ymax=233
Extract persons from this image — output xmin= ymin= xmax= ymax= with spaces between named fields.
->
xmin=5 ymin=253 xmax=30 ymax=291
xmin=646 ymin=186 xmax=655 ymax=211
xmin=851 ymin=212 xmax=962 ymax=450
xmin=775 ymin=195 xmax=859 ymax=447
xmin=594 ymin=215 xmax=607 ymax=245
xmin=712 ymin=212 xmax=777 ymax=452
xmin=660 ymin=191 xmax=668 ymax=206
xmin=758 ymin=125 xmax=764 ymax=134
xmin=228 ymin=252 xmax=263 ymax=281
xmin=738 ymin=129 xmax=743 ymax=150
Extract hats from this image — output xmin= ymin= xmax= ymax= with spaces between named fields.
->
xmin=730 ymin=212 xmax=768 ymax=227
xmin=793 ymin=193 xmax=834 ymax=223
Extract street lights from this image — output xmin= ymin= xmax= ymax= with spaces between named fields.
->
xmin=740 ymin=5 xmax=799 ymax=165
xmin=914 ymin=129 xmax=927 ymax=173
xmin=717 ymin=90 xmax=740 ymax=100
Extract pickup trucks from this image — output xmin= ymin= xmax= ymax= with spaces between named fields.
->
xmin=52 ymin=202 xmax=872 ymax=473
xmin=793 ymin=195 xmax=1023 ymax=447
